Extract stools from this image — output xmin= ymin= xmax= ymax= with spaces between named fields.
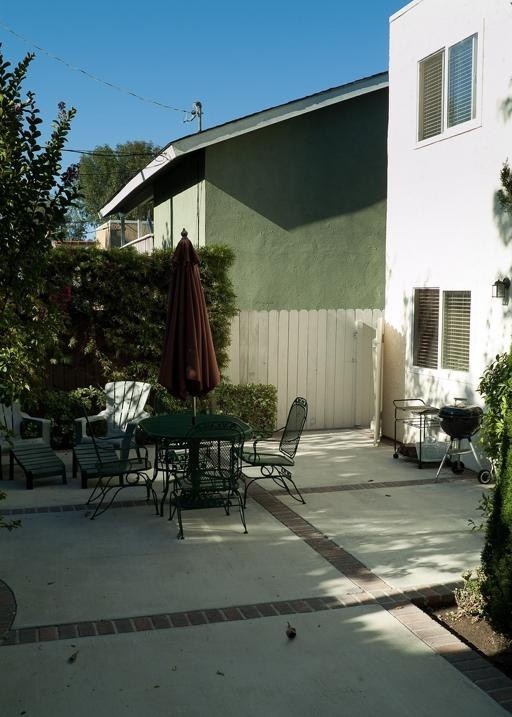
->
xmin=10 ymin=442 xmax=66 ymax=489
xmin=73 ymin=443 xmax=124 ymax=489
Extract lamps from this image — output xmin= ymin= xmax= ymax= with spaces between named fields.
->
xmin=492 ymin=278 xmax=510 ymax=298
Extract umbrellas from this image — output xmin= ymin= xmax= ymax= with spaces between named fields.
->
xmin=157 ymin=228 xmax=225 ymax=427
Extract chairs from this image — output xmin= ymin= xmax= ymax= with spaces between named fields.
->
xmin=0 ymin=395 xmax=52 ymax=466
xmin=79 ymin=389 xmax=305 ymax=539
xmin=75 ymin=380 xmax=152 ymax=463
